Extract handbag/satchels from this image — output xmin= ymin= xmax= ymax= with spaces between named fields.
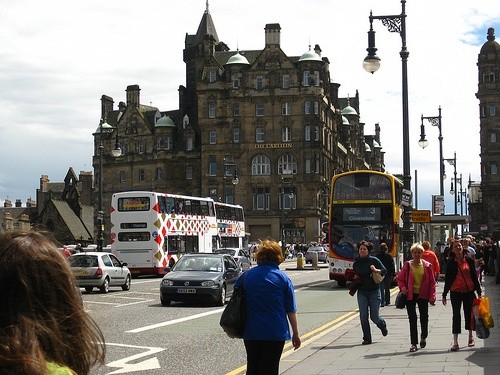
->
xmin=370 ymin=257 xmax=385 ymax=284
xmin=220 ymin=274 xmax=246 ymax=338
xmin=470 ymin=297 xmax=494 ymax=339
xmin=395 ymin=291 xmax=407 ymax=309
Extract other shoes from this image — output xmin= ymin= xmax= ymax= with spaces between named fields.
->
xmin=420 ymin=333 xmax=427 ymax=348
xmin=382 ymin=325 xmax=388 ymax=336
xmin=362 ymin=338 xmax=372 ymax=344
xmin=410 ymin=345 xmax=417 ymax=352
xmin=468 ymin=339 xmax=474 ymax=347
xmin=451 ymin=345 xmax=459 ymax=351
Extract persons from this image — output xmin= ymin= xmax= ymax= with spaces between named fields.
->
xmin=349 ymin=240 xmax=388 ymax=345
xmin=436 ymin=235 xmax=477 ymax=280
xmin=75 ymin=243 xmax=83 ymax=253
xmin=442 ymin=239 xmax=482 ymax=352
xmin=375 ymin=243 xmax=395 ymax=307
xmin=0 ymin=230 xmax=107 ymax=375
xmin=251 ymin=243 xmax=258 ymax=261
xmin=62 ymin=245 xmax=71 ymax=258
xmin=284 ymin=247 xmax=293 ymax=259
xmin=234 ymin=240 xmax=301 ymax=375
xmin=296 ymin=242 xmax=313 ymax=258
xmin=398 ymin=242 xmax=436 ymax=352
xmin=338 ymin=231 xmax=352 ymax=245
xmin=492 ymin=237 xmax=500 ymax=284
xmin=420 ymin=241 xmax=440 ymax=305
xmin=475 ymin=236 xmax=494 ymax=285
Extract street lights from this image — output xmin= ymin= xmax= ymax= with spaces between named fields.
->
xmin=96 ymin=118 xmax=123 ymax=251
xmin=362 ymin=0 xmax=415 ymax=265
xmin=444 ymin=152 xmax=472 ymax=237
xmin=280 ymin=179 xmax=295 ymax=260
xmin=419 ymin=104 xmax=445 ymax=246
xmin=222 ymin=157 xmax=239 ymax=202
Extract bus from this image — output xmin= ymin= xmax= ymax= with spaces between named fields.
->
xmin=212 ymin=202 xmax=245 ymax=257
xmin=110 ymin=190 xmax=215 ymax=278
xmin=327 ymin=170 xmax=405 ymax=286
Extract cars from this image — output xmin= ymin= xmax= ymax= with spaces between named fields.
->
xmin=159 ymin=254 xmax=242 ymax=306
xmin=305 ymin=247 xmax=328 ymax=265
xmin=214 ymin=248 xmax=252 ymax=271
xmin=67 ymin=252 xmax=132 ymax=293
